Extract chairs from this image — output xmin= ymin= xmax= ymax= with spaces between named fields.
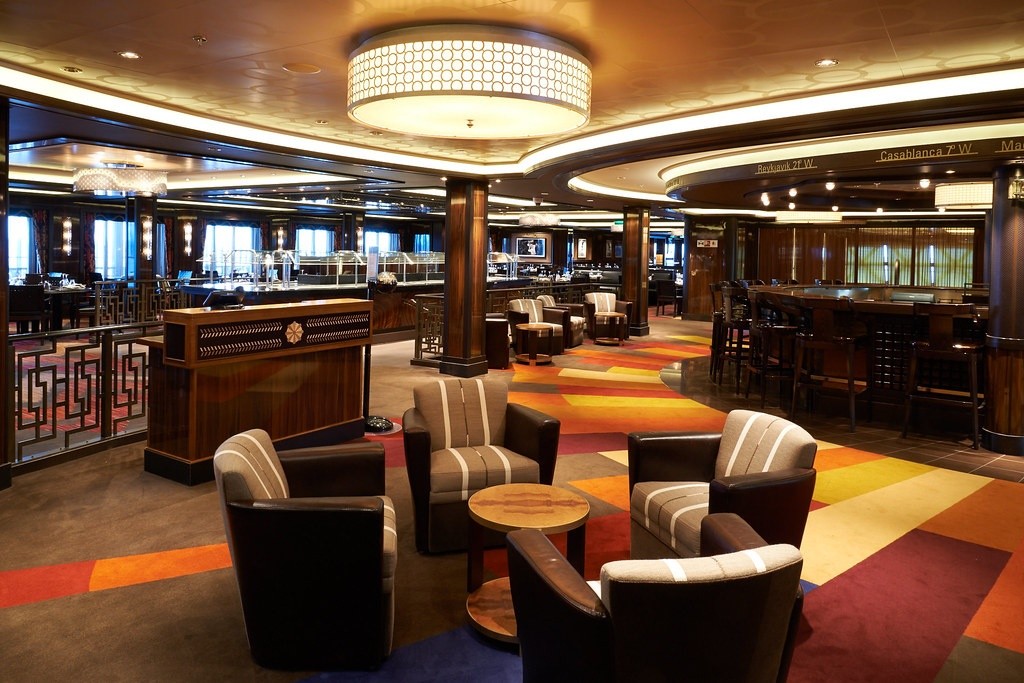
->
xmin=656 ymin=280 xmax=684 ymax=318
xmin=25 ymin=274 xmax=46 ymax=286
xmin=790 ymin=296 xmax=873 ymax=433
xmin=707 ymin=278 xmax=798 ymax=410
xmin=899 ymin=302 xmax=986 ymax=451
xmin=9 ymin=285 xmax=51 ymax=346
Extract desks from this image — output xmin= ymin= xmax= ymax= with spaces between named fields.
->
xmin=43 ymin=286 xmax=93 ymax=337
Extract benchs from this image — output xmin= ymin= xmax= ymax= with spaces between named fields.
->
xmin=649 ymin=268 xmax=676 ymax=281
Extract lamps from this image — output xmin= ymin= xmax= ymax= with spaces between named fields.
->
xmin=934 ymin=181 xmax=994 ymax=211
xmin=71 ymin=167 xmax=168 ymax=198
xmin=345 ymin=22 xmax=593 ymax=141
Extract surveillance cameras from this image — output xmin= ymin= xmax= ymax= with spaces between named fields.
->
xmin=533 ymin=198 xmax=542 ymax=206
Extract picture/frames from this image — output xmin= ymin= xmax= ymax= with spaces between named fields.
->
xmin=515 ymin=237 xmax=546 ymax=258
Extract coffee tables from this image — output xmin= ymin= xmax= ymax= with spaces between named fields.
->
xmin=515 ymin=322 xmax=554 ymax=367
xmin=593 ymin=312 xmax=625 ymax=346
xmin=464 ymin=482 xmax=591 ymax=644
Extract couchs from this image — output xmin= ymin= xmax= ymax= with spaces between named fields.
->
xmin=583 ymin=291 xmax=633 ymax=339
xmin=507 ymin=299 xmax=564 ymax=356
xmin=627 ymin=409 xmax=818 ymax=558
xmin=506 ymin=512 xmax=805 ymax=683
xmin=213 ymin=429 xmax=399 ymax=673
xmin=402 ymin=377 xmax=561 ymax=556
xmin=536 ymin=294 xmax=585 ymax=348
